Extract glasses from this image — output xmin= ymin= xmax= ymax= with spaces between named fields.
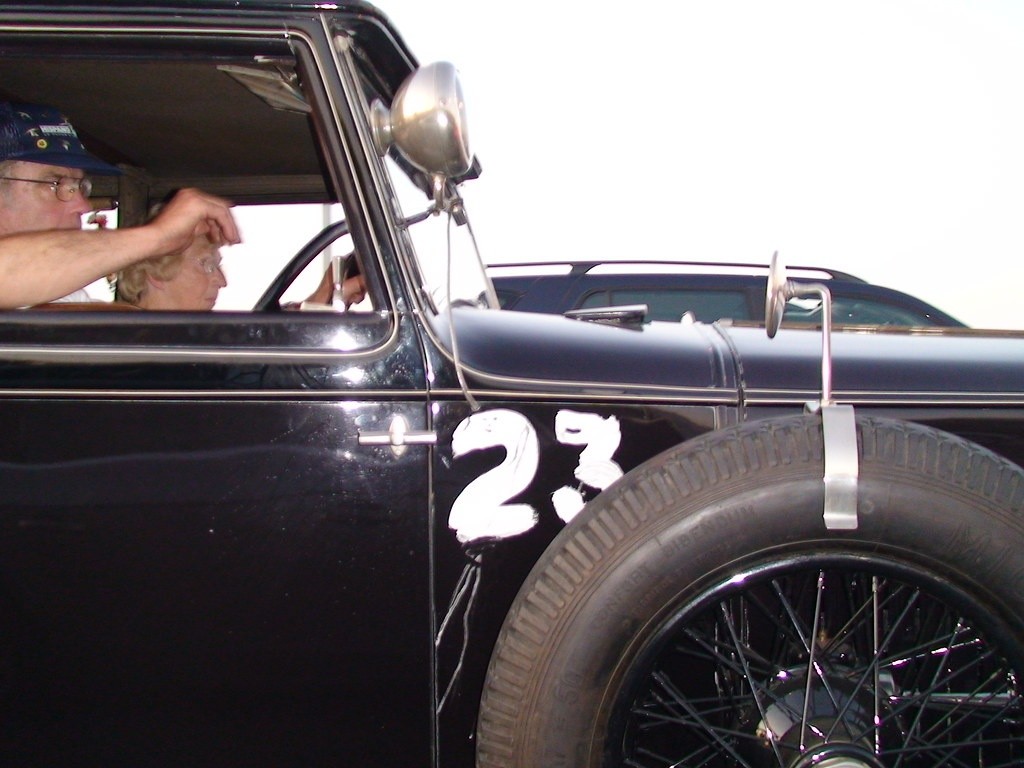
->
xmin=0 ymin=174 xmax=93 ymax=202
xmin=182 ymin=254 xmax=223 ymax=274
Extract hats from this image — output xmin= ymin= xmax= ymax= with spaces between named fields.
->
xmin=0 ymin=103 xmax=124 ymax=177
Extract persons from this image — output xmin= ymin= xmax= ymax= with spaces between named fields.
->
xmin=0 ymin=98 xmax=242 ymax=308
xmin=106 ymin=184 xmax=366 ymax=311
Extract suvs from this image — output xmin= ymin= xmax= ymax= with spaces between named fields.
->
xmin=475 ymin=260 xmax=966 ymax=334
xmin=0 ymin=1 xmax=1024 ymax=766
